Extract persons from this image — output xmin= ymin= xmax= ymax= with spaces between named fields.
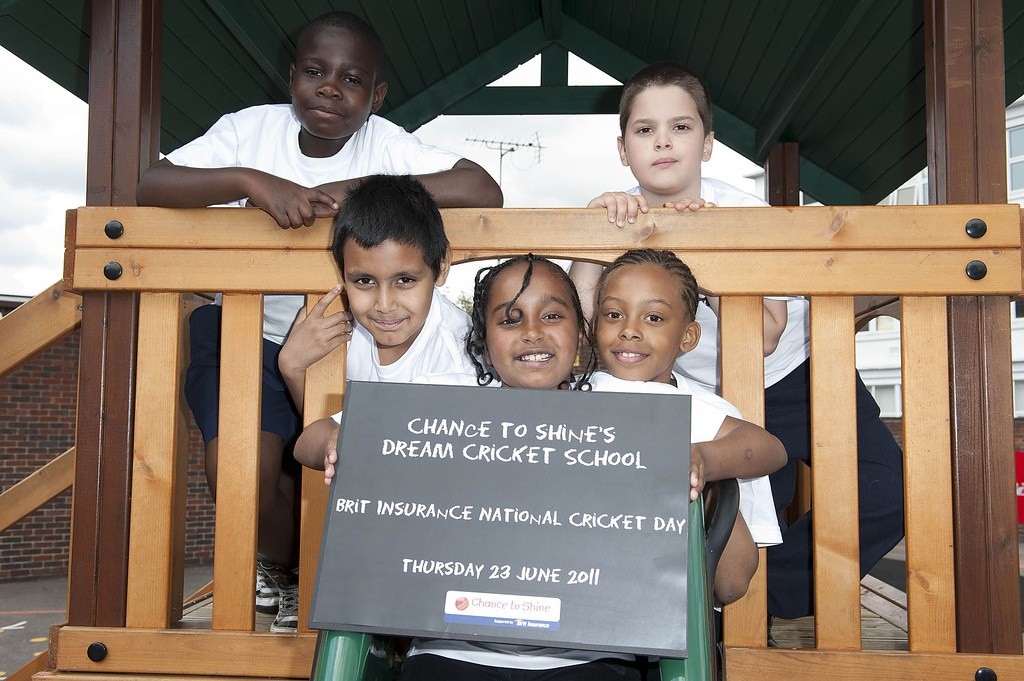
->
xmin=136 ymin=11 xmax=503 ymax=634
xmin=278 ymin=175 xmax=473 ymax=412
xmin=566 ymin=63 xmax=905 ymax=647
xmin=597 ymin=248 xmax=781 ymax=607
xmin=294 ymin=255 xmax=788 ymax=681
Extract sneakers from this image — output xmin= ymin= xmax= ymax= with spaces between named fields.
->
xmin=254 ymin=559 xmax=297 ymax=632
